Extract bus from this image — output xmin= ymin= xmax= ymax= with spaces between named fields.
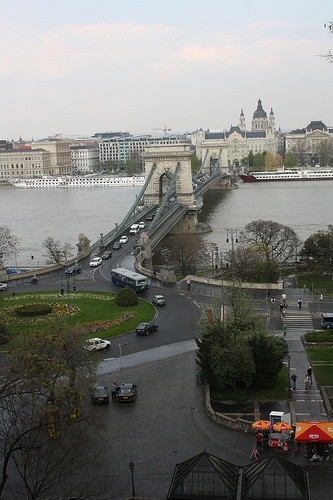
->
xmin=110 ymin=267 xmax=149 ymax=295
xmin=321 ymin=312 xmax=333 ymax=329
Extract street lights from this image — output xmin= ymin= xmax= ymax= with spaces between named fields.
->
xmin=129 ymin=460 xmax=135 ymax=497
xmin=226 ymin=228 xmax=239 ymax=279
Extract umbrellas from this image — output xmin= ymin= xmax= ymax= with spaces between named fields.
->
xmin=273 ymin=421 xmax=293 ymax=432
xmin=252 ymin=419 xmax=272 ymax=433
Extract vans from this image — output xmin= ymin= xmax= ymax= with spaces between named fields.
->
xmin=6 ymin=268 xmax=19 ymax=274
xmin=130 ymin=223 xmax=140 ymax=235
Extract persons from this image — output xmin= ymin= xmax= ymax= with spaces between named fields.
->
xmin=298 ymin=298 xmax=302 ymax=309
xmin=307 ymin=366 xmax=312 ymax=385
xmin=255 ymin=430 xmax=270 ymax=448
xmin=271 ymin=297 xmax=276 ymax=309
xmin=304 ymin=374 xmax=309 ymax=390
xmin=291 ymin=372 xmax=297 ymax=390
xmin=280 ymin=293 xmax=288 ymax=318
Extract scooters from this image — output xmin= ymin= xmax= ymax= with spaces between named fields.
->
xmin=31 ymin=278 xmax=38 ymax=283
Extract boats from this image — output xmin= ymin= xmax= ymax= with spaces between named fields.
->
xmin=7 ymin=174 xmax=146 ymax=189
xmin=238 ymin=165 xmax=333 ymax=183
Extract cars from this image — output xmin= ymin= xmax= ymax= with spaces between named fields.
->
xmin=138 ymin=221 xmax=146 ymax=229
xmin=65 ymin=265 xmax=80 ymax=276
xmin=135 ymin=323 xmax=159 ymax=336
xmin=119 ymin=235 xmax=129 ymax=244
xmin=113 ymin=242 xmax=121 ymax=250
xmin=83 ymin=337 xmax=112 ymax=353
xmin=116 ymin=382 xmax=137 ymax=403
xmin=0 ymin=283 xmax=8 ymax=291
xmin=89 ymin=256 xmax=103 ymax=267
xmin=101 ymin=250 xmax=112 ymax=260
xmin=152 ymin=294 xmax=166 ymax=306
xmin=147 ymin=195 xmax=178 ymax=221
xmin=91 ymin=385 xmax=109 ymax=406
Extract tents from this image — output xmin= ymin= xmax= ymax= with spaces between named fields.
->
xmin=294 ymin=420 xmax=333 ymax=443
